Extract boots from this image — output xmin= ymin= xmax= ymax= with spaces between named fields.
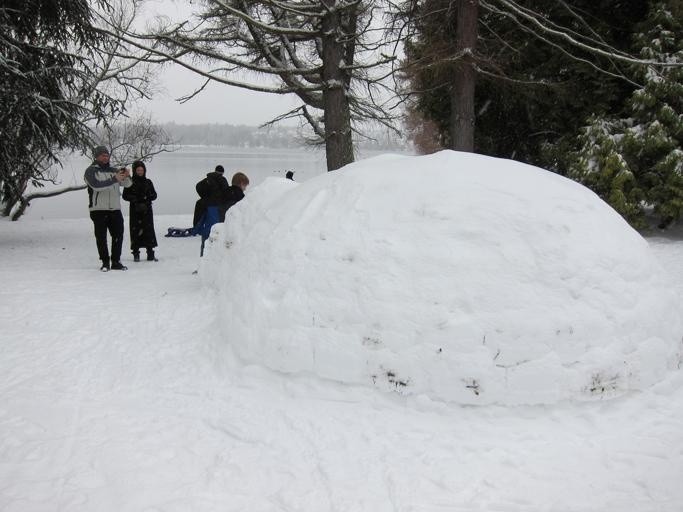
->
xmin=131 ymin=251 xmax=142 ymax=263
xmin=144 ymin=250 xmax=159 ymax=263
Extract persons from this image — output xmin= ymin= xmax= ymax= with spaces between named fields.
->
xmin=121 ymin=159 xmax=159 ymax=263
xmin=192 ymin=165 xmax=229 ymax=226
xmin=199 ymin=170 xmax=249 ymax=260
xmin=83 ymin=145 xmax=134 ymax=272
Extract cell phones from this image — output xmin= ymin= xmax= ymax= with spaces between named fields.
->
xmin=121 ymin=168 xmax=126 ymax=173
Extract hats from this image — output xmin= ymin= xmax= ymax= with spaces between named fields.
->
xmin=93 ymin=145 xmax=109 ymax=158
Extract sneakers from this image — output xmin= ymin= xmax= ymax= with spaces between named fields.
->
xmin=110 ymin=262 xmax=127 ymax=272
xmin=100 ymin=262 xmax=110 ymax=271
